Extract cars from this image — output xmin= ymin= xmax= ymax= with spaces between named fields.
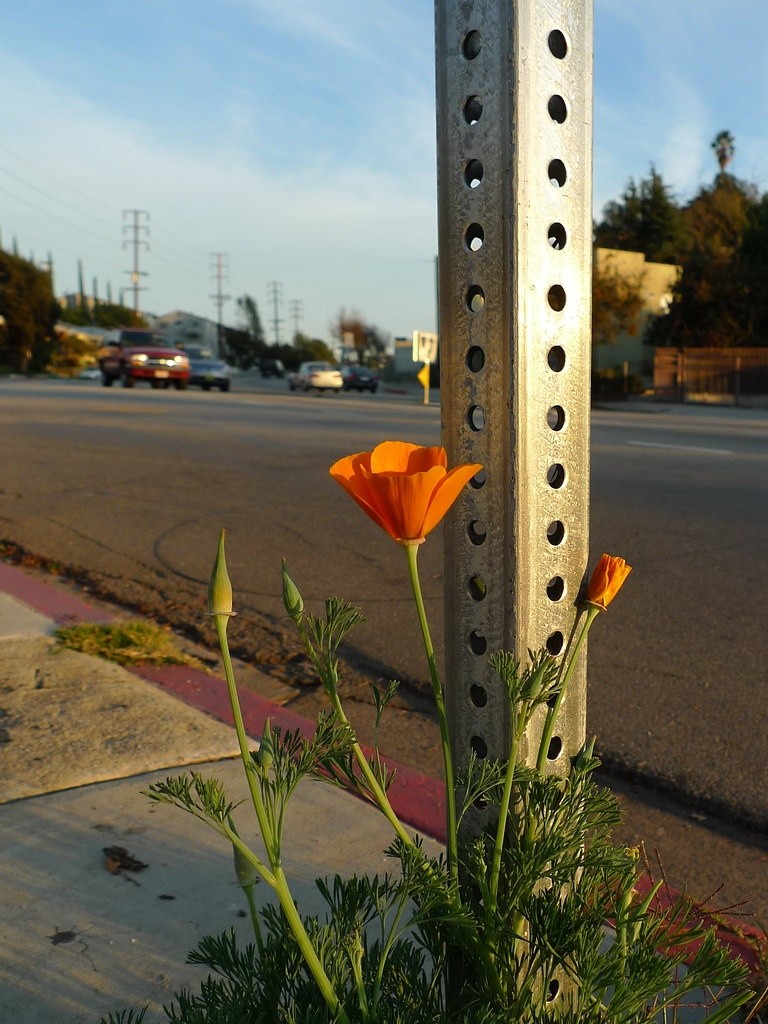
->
xmin=289 ymin=361 xmax=343 ymax=394
xmin=151 ymin=342 xmax=231 ymax=392
xmin=340 ymin=364 xmax=378 ymax=394
xmin=95 ymin=330 xmax=190 ymax=391
